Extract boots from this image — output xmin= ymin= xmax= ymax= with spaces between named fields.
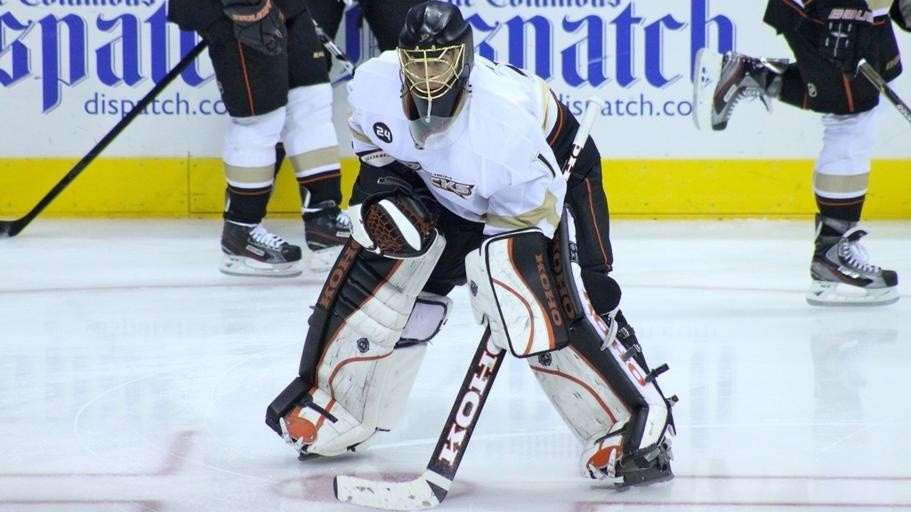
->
xmin=301 ymin=205 xmax=354 ymax=251
xmin=710 ymin=49 xmax=789 ymax=132
xmin=808 ymin=212 xmax=899 ymax=292
xmin=220 ymin=218 xmax=301 ymax=266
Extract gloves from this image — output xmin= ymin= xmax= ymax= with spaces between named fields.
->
xmin=817 ymin=6 xmax=873 ymax=78
xmin=223 ymin=1 xmax=288 ymax=55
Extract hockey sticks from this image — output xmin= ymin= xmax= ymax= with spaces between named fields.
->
xmin=0 ymin=39 xmax=208 ymax=237
xmin=334 ymin=101 xmax=600 ymax=509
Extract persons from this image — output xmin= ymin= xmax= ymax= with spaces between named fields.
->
xmin=708 ymin=0 xmax=911 ymax=305
xmin=267 ymin=2 xmax=682 ymax=489
xmin=163 ymin=2 xmax=446 ymax=281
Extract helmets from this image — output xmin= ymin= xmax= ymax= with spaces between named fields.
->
xmin=396 ymin=2 xmax=476 ymax=129
